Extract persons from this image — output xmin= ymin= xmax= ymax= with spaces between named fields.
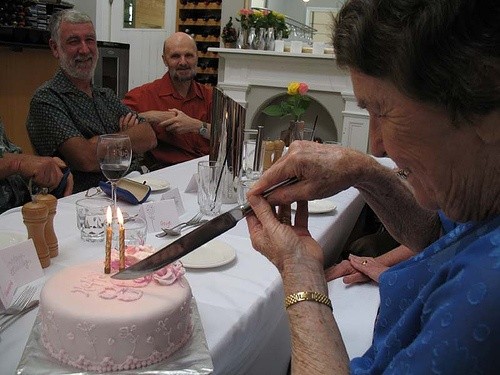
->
xmin=26 ymin=9 xmax=160 ymax=192
xmin=0 ymin=121 xmax=80 ymax=215
xmin=245 ymin=0 xmax=500 ymax=375
xmin=124 ymin=32 xmax=213 ymax=168
xmin=324 ymin=231 xmax=416 ymax=284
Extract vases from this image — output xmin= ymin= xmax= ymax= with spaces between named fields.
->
xmin=241 ymin=28 xmax=251 ymax=49
xmin=266 ymin=28 xmax=276 ymax=50
xmin=286 ymin=121 xmax=304 ymax=146
xmin=252 ymin=28 xmax=265 ymax=49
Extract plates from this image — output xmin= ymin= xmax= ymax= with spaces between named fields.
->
xmin=133 ymin=176 xmax=170 ymax=192
xmin=178 ymin=239 xmax=236 ymax=268
xmin=291 ymin=199 xmax=337 ymax=213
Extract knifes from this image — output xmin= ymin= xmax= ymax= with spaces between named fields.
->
xmin=110 ymin=177 xmax=301 ymax=280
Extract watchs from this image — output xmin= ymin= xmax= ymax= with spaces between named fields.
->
xmin=199 ymin=122 xmax=208 ymax=138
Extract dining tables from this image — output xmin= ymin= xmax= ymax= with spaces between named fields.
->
xmin=0 ymin=141 xmax=395 ymax=375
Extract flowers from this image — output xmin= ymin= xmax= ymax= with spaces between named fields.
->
xmin=262 ymin=81 xmax=310 ymax=121
xmin=235 ymin=8 xmax=289 ymax=39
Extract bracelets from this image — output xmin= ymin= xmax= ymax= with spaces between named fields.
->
xmin=285 ymin=292 xmax=333 ymax=311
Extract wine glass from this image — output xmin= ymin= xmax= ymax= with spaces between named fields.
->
xmin=97 ymin=134 xmax=132 ymax=206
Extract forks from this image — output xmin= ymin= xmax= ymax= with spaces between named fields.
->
xmin=155 ymin=211 xmax=207 ymax=238
xmin=0 ymin=285 xmax=37 ymax=315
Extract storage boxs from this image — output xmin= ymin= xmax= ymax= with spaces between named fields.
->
xmin=99 ymin=178 xmax=151 ymax=204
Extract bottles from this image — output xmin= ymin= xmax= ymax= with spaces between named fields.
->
xmin=248 ymin=27 xmax=256 ymax=50
xmin=180 ymin=0 xmax=222 ymax=86
xmin=265 ymin=27 xmax=275 ymax=51
xmin=256 ymin=27 xmax=265 ymax=50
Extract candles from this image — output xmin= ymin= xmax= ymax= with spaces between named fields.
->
xmin=105 ymin=206 xmax=112 ymax=274
xmin=116 ymin=207 xmax=125 ymax=268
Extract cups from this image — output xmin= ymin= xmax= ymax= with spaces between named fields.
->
xmin=197 ymin=161 xmax=226 ymax=216
xmin=75 ymin=197 xmax=114 ymax=242
xmin=209 ymin=87 xmax=247 ymax=204
xmin=302 ymin=128 xmax=314 ymax=140
xmin=104 ymin=216 xmax=148 ymax=250
xmin=246 ymin=140 xmax=263 ymax=173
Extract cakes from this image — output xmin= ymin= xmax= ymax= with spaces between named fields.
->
xmin=38 ymin=243 xmax=193 ymax=373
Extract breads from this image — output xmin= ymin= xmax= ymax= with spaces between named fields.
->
xmin=183 ymin=1 xmax=222 ymax=74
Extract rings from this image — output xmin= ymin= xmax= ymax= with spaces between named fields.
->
xmin=363 ymin=260 xmax=367 ymax=264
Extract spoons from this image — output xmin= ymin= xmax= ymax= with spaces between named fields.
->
xmin=161 ymin=219 xmax=209 ymax=236
